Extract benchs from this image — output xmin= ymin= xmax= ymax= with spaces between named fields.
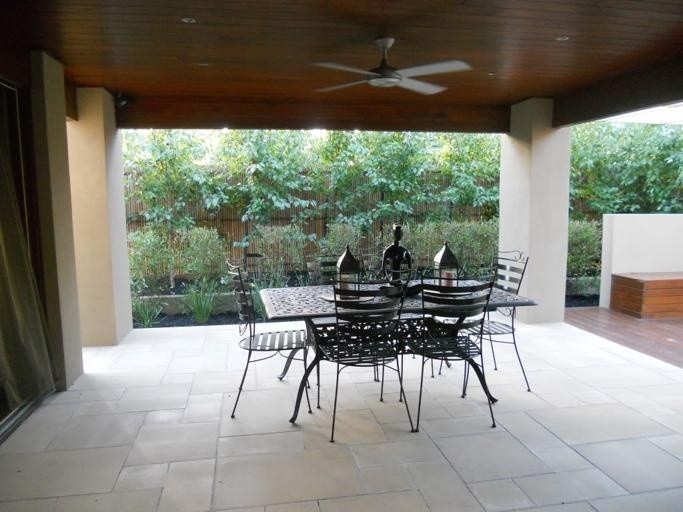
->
xmin=609 ymin=272 xmax=683 ymax=320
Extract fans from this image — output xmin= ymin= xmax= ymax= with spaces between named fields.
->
xmin=309 ymin=37 xmax=474 ymax=97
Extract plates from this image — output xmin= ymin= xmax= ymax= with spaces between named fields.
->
xmin=423 ymin=289 xmax=473 ymax=296
xmin=323 ymin=294 xmax=375 ymax=302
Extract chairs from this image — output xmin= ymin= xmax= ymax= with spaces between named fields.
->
xmin=223 ymin=260 xmax=316 ymax=420
xmin=313 ymin=275 xmax=415 ymax=444
xmin=301 ymin=247 xmax=383 ymax=405
xmin=437 ymin=245 xmax=534 ymax=398
xmin=398 ymin=265 xmax=498 ymax=436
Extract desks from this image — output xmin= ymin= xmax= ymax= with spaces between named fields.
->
xmin=257 ymin=276 xmax=535 ymax=431
